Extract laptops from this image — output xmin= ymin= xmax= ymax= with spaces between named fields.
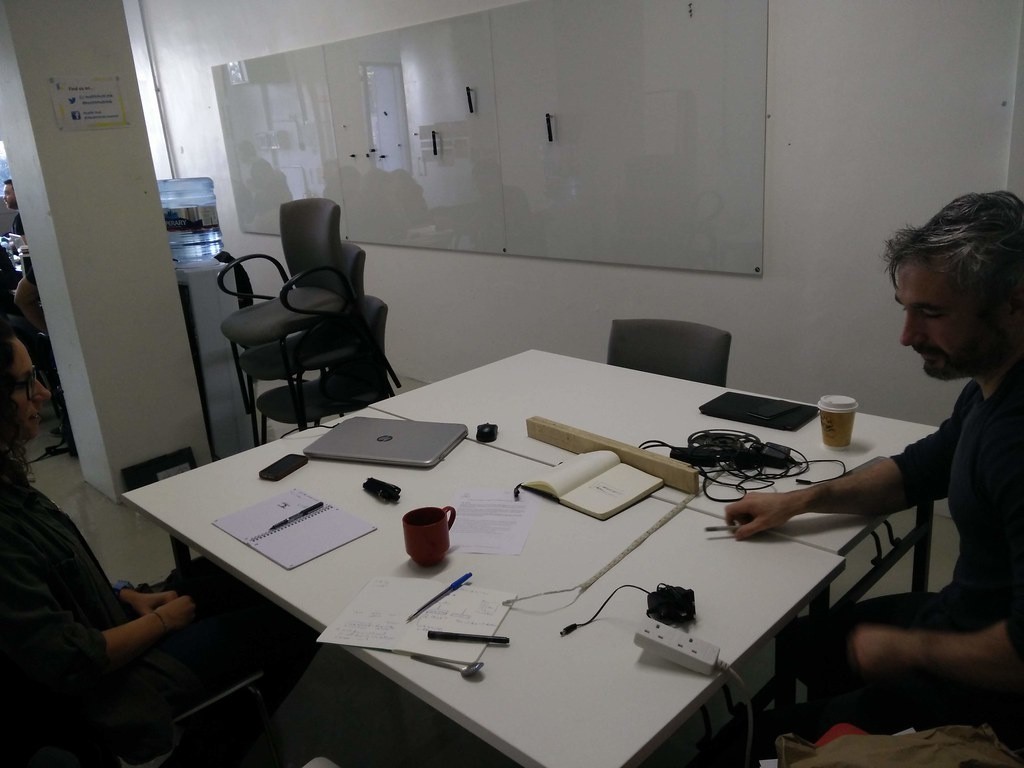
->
xmin=699 ymin=393 xmax=820 ymax=431
xmin=304 ymin=417 xmax=468 ymax=468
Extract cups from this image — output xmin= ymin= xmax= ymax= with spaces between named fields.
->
xmin=403 ymin=506 xmax=456 ymax=566
xmin=817 ymin=395 xmax=859 ymax=449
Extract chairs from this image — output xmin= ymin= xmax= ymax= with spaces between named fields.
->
xmin=213 ymin=197 xmax=403 ymax=445
xmin=609 ymin=318 xmax=732 ymax=392
xmin=31 ymin=671 xmax=284 ymax=767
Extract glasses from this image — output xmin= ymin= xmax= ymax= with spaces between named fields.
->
xmin=16 ymin=365 xmax=37 ymax=400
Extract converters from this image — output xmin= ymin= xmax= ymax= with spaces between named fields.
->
xmin=669 ymin=447 xmax=718 ymax=467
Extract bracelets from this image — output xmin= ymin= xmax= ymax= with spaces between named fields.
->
xmin=152 ymin=612 xmax=167 ymax=633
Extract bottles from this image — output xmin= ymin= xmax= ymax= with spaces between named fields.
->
xmin=157 ymin=177 xmax=224 ymax=262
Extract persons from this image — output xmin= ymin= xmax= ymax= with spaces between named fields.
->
xmin=0 ymin=179 xmax=65 ymax=438
xmin=0 ymin=329 xmax=322 ymax=768
xmin=725 ymin=190 xmax=1024 ymax=752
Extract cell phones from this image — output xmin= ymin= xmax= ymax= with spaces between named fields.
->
xmin=747 ymin=400 xmax=800 ymax=420
xmin=259 ymin=454 xmax=308 ymax=482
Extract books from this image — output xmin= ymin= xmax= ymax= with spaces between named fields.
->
xmin=513 ymin=450 xmax=664 ymax=521
xmin=211 ymin=487 xmax=377 ymax=570
xmin=316 ymin=576 xmax=518 ymax=665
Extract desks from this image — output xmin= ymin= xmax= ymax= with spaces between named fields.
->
xmin=123 ymin=351 xmax=938 ymax=768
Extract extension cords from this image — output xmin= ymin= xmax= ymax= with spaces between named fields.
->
xmin=634 ymin=619 xmax=720 ymax=676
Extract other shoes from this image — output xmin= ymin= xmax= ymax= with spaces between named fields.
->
xmin=48 ymin=424 xmax=61 ymax=436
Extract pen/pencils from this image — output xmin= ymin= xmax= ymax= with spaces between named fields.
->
xmin=269 ymin=501 xmax=324 ymax=531
xmin=705 ymin=525 xmax=740 ymax=531
xmin=427 ymin=630 xmax=510 ymax=644
xmin=406 ymin=571 xmax=473 ymax=622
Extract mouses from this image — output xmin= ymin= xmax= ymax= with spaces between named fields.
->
xmin=476 ymin=423 xmax=498 ymax=442
